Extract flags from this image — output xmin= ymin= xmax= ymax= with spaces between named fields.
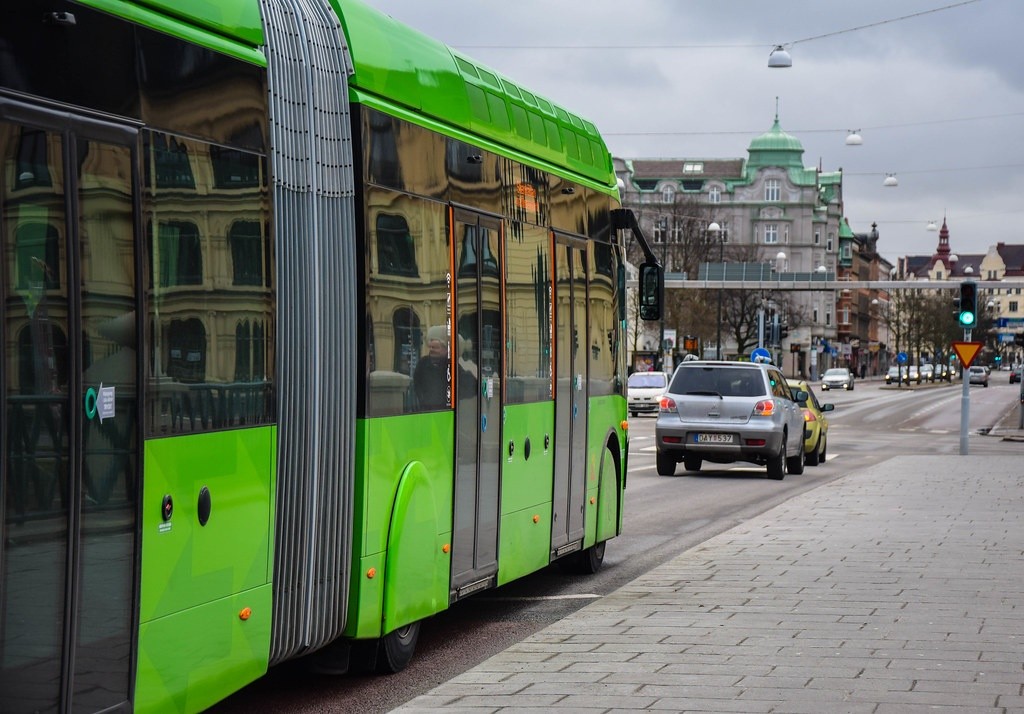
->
xmin=28 ymin=260 xmax=51 ymax=317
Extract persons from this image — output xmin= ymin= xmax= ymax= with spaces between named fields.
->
xmin=413 ymin=338 xmax=476 ymax=414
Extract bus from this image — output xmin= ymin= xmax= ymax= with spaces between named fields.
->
xmin=0 ymin=0 xmax=665 ymax=714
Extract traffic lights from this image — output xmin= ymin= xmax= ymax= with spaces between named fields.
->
xmin=959 ymin=282 xmax=976 ymax=326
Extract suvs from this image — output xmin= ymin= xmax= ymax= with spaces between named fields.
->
xmin=654 ymin=354 xmax=809 ymax=480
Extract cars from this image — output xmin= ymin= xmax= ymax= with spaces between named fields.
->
xmin=969 ymin=366 xmax=991 ymax=387
xmin=785 ymin=379 xmax=835 ymax=466
xmin=885 ymin=363 xmax=956 ymax=386
xmin=820 ymin=368 xmax=854 ymax=391
xmin=628 ymin=372 xmax=674 ymax=417
xmin=1009 ymin=364 xmax=1022 ymax=384
